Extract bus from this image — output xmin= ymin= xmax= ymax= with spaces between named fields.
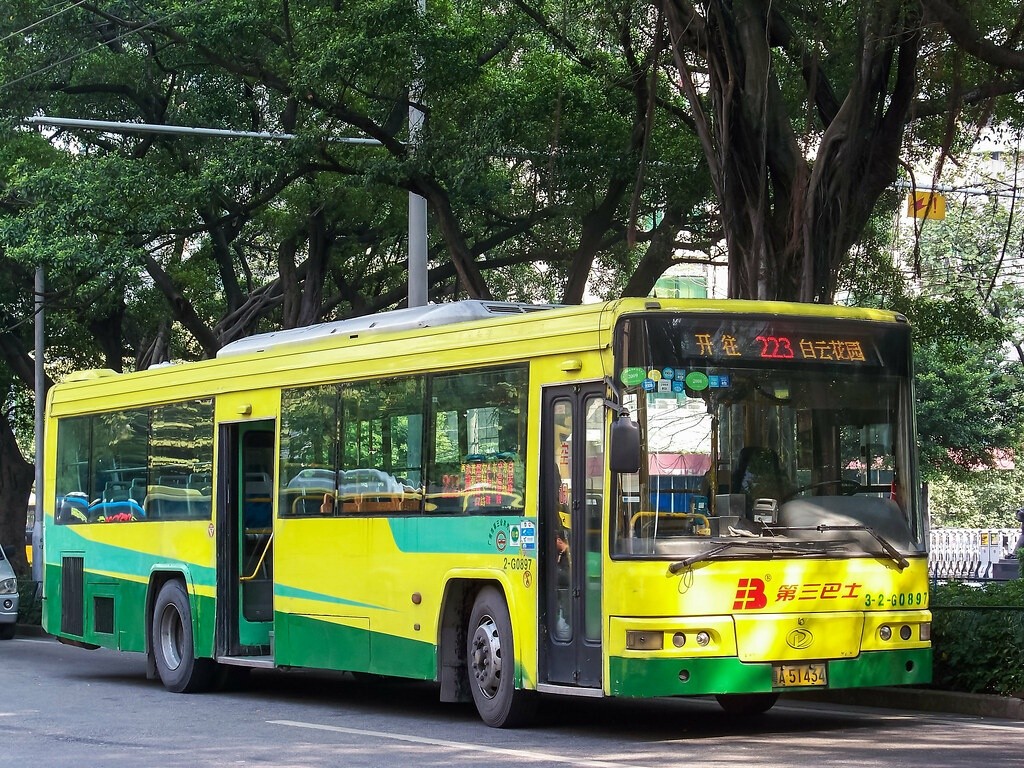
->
xmin=39 ymin=297 xmax=936 ymax=731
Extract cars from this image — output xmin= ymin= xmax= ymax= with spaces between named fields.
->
xmin=0 ymin=545 xmax=20 ymax=639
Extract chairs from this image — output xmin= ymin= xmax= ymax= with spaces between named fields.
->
xmin=734 ymin=446 xmax=794 ymax=515
xmin=102 ymin=452 xmax=523 ymax=518
